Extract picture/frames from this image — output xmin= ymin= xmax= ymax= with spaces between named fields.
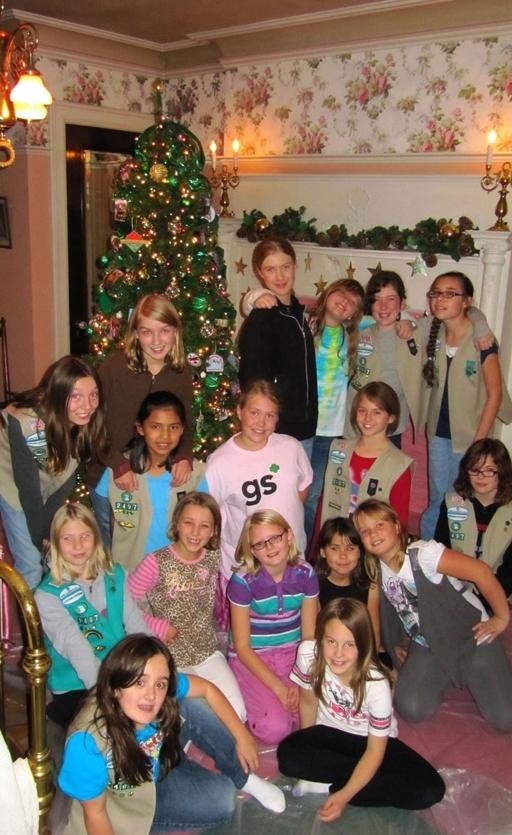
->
xmin=0 ymin=196 xmax=12 ymax=248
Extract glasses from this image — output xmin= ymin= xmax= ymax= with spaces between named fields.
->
xmin=428 ymin=289 xmax=466 ymax=299
xmin=467 ymin=469 xmax=499 ymax=477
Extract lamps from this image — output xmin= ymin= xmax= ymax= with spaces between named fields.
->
xmin=0 ymin=0 xmax=54 ymax=169
xmin=480 ymin=129 xmax=512 ymax=231
xmin=207 ymin=138 xmax=240 ymax=218
